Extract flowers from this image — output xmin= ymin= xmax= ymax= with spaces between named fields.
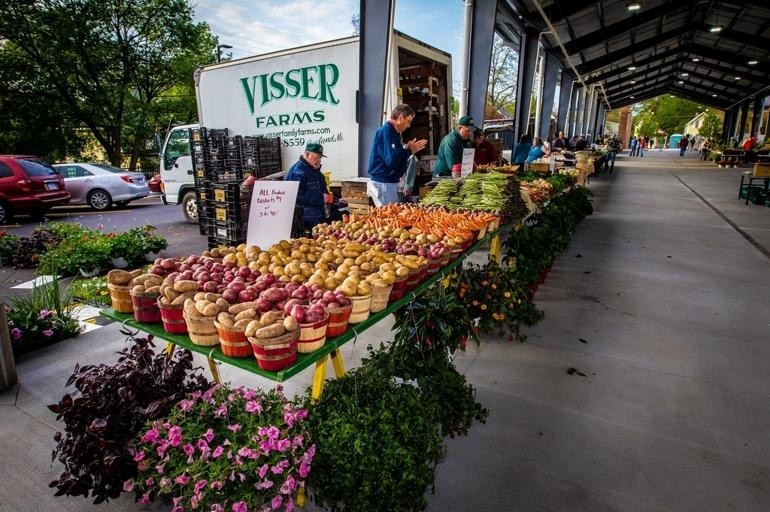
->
xmin=381 ymin=251 xmax=548 ymax=363
xmin=0 ymin=264 xmax=87 ymax=356
xmin=115 ymin=378 xmax=319 ymax=511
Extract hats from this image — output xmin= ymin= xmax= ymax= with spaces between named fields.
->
xmin=306 ymin=144 xmax=327 ymax=157
xmin=459 ymin=117 xmax=478 ymax=129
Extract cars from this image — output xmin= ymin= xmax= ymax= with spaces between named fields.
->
xmin=59 ymin=158 xmax=148 ymax=211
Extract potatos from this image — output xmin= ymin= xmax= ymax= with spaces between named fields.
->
xmin=108 ymin=268 xmax=298 ymax=339
xmin=313 ymin=219 xmax=462 ymax=250
xmin=204 ymin=237 xmax=428 ymax=296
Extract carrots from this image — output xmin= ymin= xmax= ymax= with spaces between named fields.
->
xmin=342 ymin=202 xmax=495 ymax=240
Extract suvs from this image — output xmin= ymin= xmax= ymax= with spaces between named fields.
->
xmin=0 ymin=151 xmax=67 ymax=220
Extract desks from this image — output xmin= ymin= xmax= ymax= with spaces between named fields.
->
xmin=97 ymin=229 xmax=503 ymax=508
xmin=736 ymin=175 xmax=769 ymax=203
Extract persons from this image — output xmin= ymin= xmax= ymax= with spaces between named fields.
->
xmin=434 ymin=115 xmax=474 ymax=177
xmin=472 ymin=129 xmax=502 ymax=165
xmin=658 ymin=134 xmax=665 ymax=153
xmin=511 ymin=129 xmax=654 ymax=176
xmin=396 ymin=139 xmax=419 ymax=204
xmin=677 ymin=133 xmax=770 ymax=164
xmin=286 ymin=142 xmax=334 ymax=233
xmin=366 ymin=104 xmax=427 ymax=208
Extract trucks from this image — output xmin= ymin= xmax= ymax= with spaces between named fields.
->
xmin=482 ymin=119 xmax=517 ymax=169
xmin=155 ymin=29 xmax=457 ymax=212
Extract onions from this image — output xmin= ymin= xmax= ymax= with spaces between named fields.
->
xmin=148 ymin=254 xmax=350 ymax=323
xmin=323 ymin=227 xmax=448 ymax=262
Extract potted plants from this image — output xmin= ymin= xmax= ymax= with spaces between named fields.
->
xmin=32 ymin=216 xmax=172 ymax=278
